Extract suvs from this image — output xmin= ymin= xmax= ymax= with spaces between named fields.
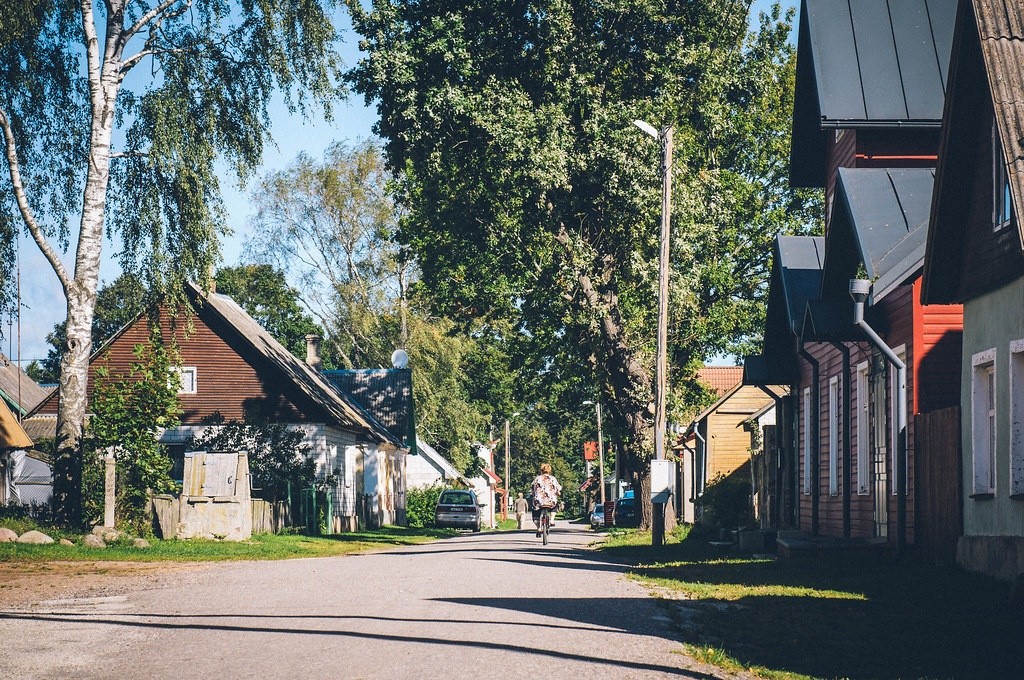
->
xmin=434 ymin=488 xmax=484 ymax=532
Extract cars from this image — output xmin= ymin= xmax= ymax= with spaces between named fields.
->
xmin=615 ymin=489 xmax=642 ymax=528
xmin=589 ymin=503 xmax=605 ymax=530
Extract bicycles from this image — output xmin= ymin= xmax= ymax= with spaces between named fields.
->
xmin=532 ymin=485 xmax=555 ymax=545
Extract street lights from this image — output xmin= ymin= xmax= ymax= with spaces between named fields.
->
xmin=505 ymin=411 xmax=522 ymax=490
xmin=633 ymin=117 xmax=673 ymax=549
xmin=583 ymin=400 xmax=606 ymax=505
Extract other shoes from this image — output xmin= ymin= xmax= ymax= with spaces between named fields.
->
xmin=536 ymin=529 xmax=541 ymax=537
xmin=550 ymin=522 xmax=555 ymax=526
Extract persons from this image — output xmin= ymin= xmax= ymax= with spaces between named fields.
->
xmin=514 ymin=493 xmax=528 ymax=529
xmin=531 ymin=464 xmax=562 ymax=538
